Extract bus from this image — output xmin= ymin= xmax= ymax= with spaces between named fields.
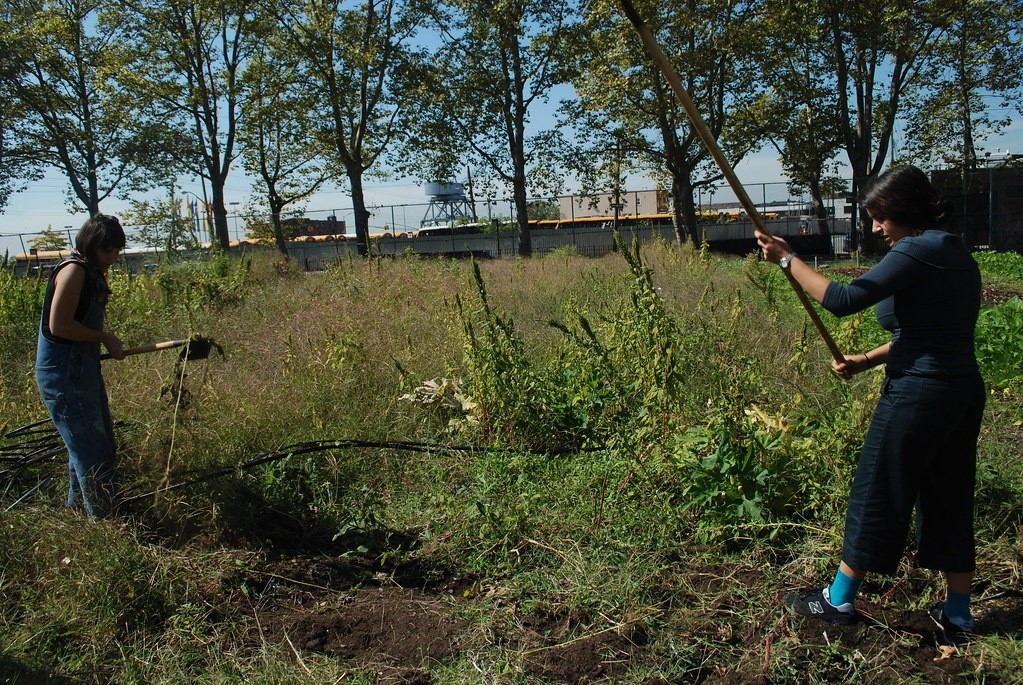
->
xmin=415 ymin=211 xmax=779 ymax=236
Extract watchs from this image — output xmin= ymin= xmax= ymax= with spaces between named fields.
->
xmin=778 ymin=251 xmax=797 ymax=269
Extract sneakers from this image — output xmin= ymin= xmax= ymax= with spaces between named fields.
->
xmin=898 ymin=602 xmax=978 ymax=646
xmin=783 ymin=584 xmax=857 ymax=627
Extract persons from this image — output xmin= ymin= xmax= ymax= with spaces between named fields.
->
xmin=33 ymin=213 xmax=127 ymax=520
xmin=753 ymin=164 xmax=987 ymax=641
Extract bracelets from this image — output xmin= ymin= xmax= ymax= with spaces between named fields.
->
xmin=863 ymin=354 xmax=871 ymax=369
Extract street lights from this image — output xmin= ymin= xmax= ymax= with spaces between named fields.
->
xmin=181 ymin=166 xmax=214 ymax=245
